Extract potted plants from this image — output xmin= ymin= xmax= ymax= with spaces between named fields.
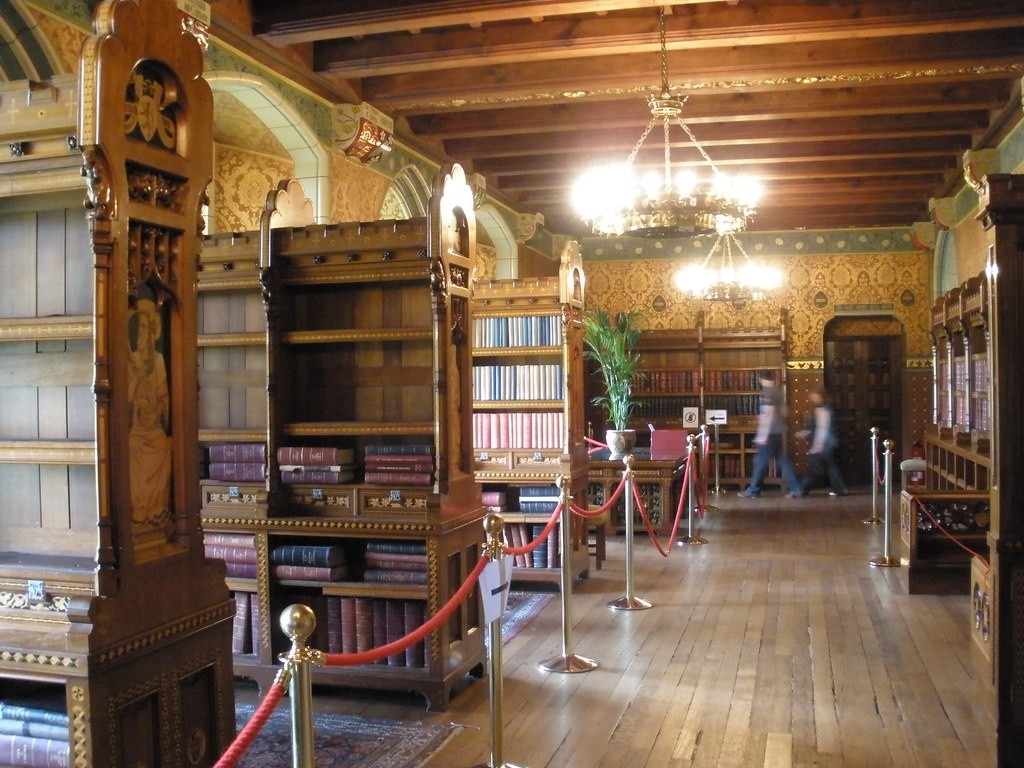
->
xmin=572 ymin=305 xmax=645 ymax=454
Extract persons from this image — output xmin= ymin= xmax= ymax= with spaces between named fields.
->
xmin=737 ymin=374 xmax=802 ymax=499
xmin=794 ymin=386 xmax=849 ymax=496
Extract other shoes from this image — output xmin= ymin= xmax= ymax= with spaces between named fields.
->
xmin=829 ymin=489 xmax=849 ymax=496
xmin=785 ymin=491 xmax=804 ymax=498
xmin=737 ymin=491 xmax=761 ymax=499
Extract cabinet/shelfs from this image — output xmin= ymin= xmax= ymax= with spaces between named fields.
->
xmin=192 ymin=160 xmax=487 ymax=715
xmin=0 ymin=0 xmax=234 ymax=768
xmin=466 ymin=239 xmax=589 ymax=591
xmin=598 ymin=307 xmax=789 ymax=494
xmin=900 ymin=270 xmax=995 ymax=595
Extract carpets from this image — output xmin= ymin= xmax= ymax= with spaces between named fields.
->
xmin=485 ymin=591 xmax=555 ymax=649
xmin=234 ymin=703 xmax=462 ymax=768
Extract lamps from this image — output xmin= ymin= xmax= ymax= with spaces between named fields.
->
xmin=670 ymin=233 xmax=784 ymax=303
xmin=564 ymin=5 xmax=766 ymax=240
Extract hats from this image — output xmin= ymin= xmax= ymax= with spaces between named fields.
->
xmin=761 ymin=372 xmax=773 ymax=381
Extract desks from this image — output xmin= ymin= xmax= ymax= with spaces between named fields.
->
xmin=588 ymin=446 xmax=700 ymax=539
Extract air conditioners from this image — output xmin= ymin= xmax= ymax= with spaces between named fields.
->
xmin=588 ymin=503 xmax=607 ymax=571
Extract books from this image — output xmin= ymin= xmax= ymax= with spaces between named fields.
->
xmin=482 ymin=486 xmax=561 ymax=568
xmin=472 ymin=316 xmax=562 ymax=348
xmin=627 ymin=370 xmax=782 ymax=479
xmin=196 ymin=442 xmax=434 ymax=668
xmin=473 ymin=413 xmax=563 ymax=449
xmin=473 ymin=365 xmax=562 ymax=401
xmin=0 ymin=690 xmax=70 ymax=768
xmin=938 ymin=358 xmax=992 ymax=432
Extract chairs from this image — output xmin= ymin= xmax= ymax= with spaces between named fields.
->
xmin=651 ymin=428 xmax=688 ymax=447
